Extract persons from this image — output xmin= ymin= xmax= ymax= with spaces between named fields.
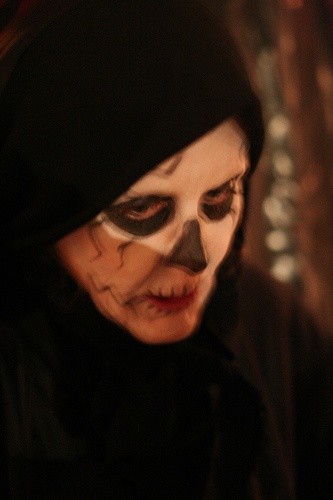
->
xmin=3 ymin=0 xmax=333 ymax=499
